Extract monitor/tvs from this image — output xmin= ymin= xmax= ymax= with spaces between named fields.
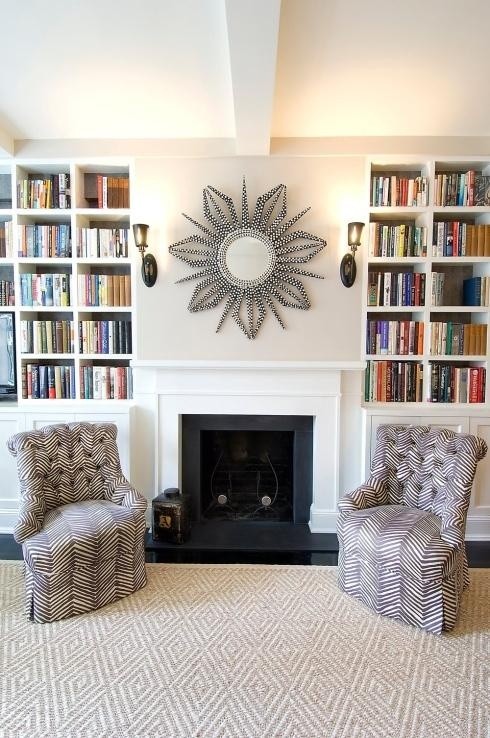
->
xmin=0 ymin=312 xmax=17 ymax=394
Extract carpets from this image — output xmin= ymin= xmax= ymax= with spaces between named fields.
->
xmin=0 ymin=560 xmax=489 ymax=737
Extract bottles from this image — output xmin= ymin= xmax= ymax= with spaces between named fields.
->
xmin=150 ymin=488 xmax=191 ymax=544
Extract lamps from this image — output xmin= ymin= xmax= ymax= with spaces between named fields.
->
xmin=340 ymin=222 xmax=365 ymax=288
xmin=133 ymin=223 xmax=158 ymax=287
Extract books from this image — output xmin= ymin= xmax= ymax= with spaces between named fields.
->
xmin=1 ymin=171 xmax=133 ymax=402
xmin=364 ymin=167 xmax=490 ymax=403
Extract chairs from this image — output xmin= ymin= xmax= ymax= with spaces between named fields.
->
xmin=5 ymin=422 xmax=148 ymax=625
xmin=336 ymin=423 xmax=488 ymax=636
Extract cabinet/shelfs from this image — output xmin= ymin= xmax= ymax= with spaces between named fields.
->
xmin=0 ymin=406 xmax=139 ymax=535
xmin=363 ymin=403 xmax=490 ymax=541
xmin=362 ymin=154 xmax=490 ymax=417
xmin=0 ymin=160 xmax=138 ymax=412
